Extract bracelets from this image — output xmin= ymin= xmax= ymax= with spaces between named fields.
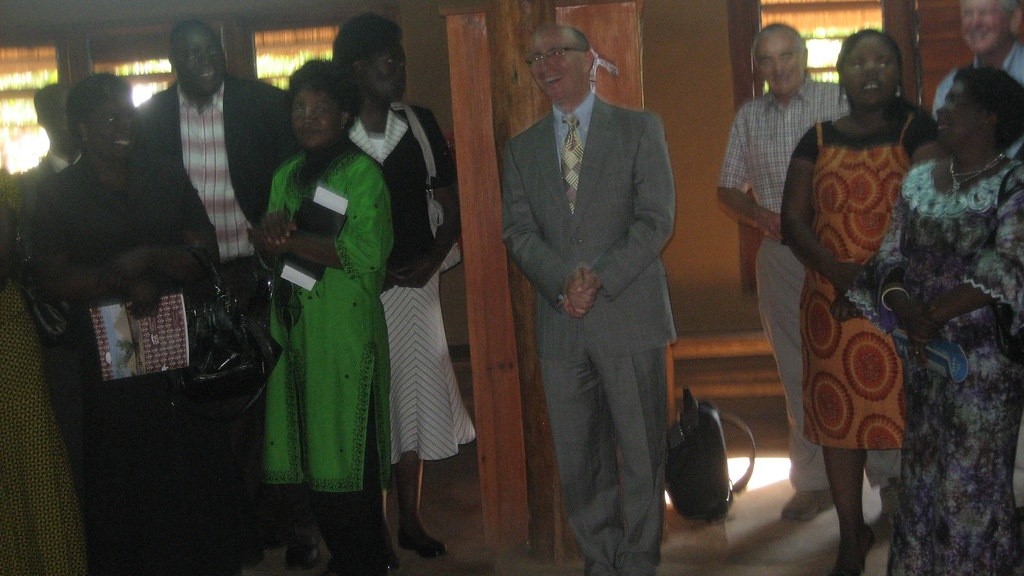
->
xmin=881 ymin=287 xmax=911 ymax=312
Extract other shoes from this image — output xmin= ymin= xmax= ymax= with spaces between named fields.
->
xmin=782 ymin=489 xmax=836 ymax=521
xmin=876 ymin=485 xmax=899 ymax=522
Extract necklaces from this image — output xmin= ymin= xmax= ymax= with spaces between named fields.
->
xmin=945 ymin=152 xmax=1007 ymax=197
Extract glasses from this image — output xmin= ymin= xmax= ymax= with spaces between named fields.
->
xmin=525 ymin=48 xmax=587 ymax=64
xmin=82 ymin=108 xmax=136 ymax=126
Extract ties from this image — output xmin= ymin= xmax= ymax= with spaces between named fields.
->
xmin=560 ymin=115 xmax=585 ymax=214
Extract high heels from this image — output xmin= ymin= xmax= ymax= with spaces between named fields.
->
xmin=829 ymin=525 xmax=875 ymax=576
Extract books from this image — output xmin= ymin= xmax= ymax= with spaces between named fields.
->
xmin=89 ymin=293 xmax=190 ymax=383
xmin=283 ymin=198 xmax=347 ymax=283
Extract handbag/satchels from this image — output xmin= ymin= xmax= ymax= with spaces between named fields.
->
xmin=177 ymin=247 xmax=282 ymax=381
xmin=402 ymin=105 xmax=461 ymax=273
xmin=12 ymin=276 xmax=68 ymax=347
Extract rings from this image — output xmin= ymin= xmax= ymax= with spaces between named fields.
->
xmin=124 ymin=301 xmax=132 ymax=307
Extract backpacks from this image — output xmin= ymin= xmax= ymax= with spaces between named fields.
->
xmin=664 ymin=386 xmax=755 ymax=521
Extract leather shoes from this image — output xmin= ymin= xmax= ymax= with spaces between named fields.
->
xmin=387 ymin=550 xmax=398 ymax=569
xmin=398 ymin=526 xmax=446 ymax=558
xmin=285 ymin=531 xmax=320 ymax=571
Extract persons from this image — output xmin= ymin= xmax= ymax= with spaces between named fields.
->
xmin=144 ymin=20 xmax=300 ymax=263
xmin=24 ymin=84 xmax=85 ymax=192
xmin=498 ymin=23 xmax=676 ymax=576
xmin=716 ymin=23 xmax=904 ymax=523
xmin=780 ymin=28 xmax=937 ymax=576
xmin=247 ymin=58 xmax=393 ymax=576
xmin=932 ymin=0 xmax=1024 ymax=160
xmin=332 ymin=12 xmax=477 ymax=558
xmin=843 ymin=65 xmax=1024 ymax=576
xmin=22 ymin=73 xmax=262 ymax=576
